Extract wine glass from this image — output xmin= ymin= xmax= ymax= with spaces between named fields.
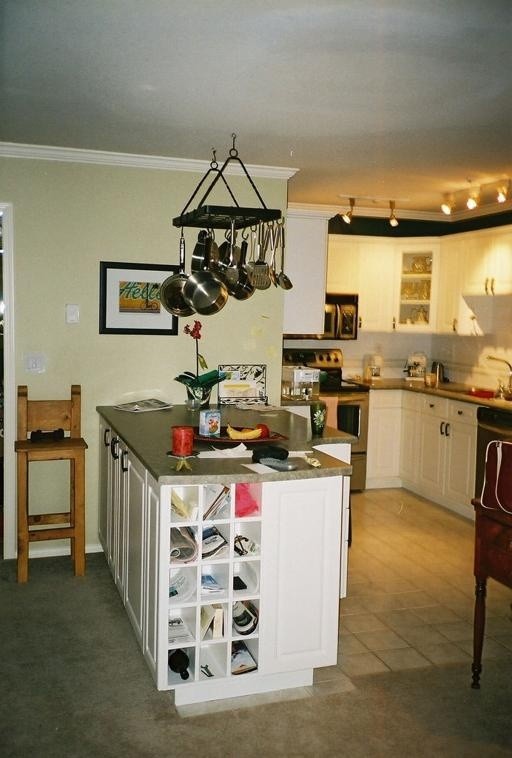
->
xmin=419 ymin=280 xmax=430 ymax=300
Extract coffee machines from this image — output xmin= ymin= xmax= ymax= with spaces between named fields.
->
xmin=363 ymin=354 xmax=384 ymax=381
xmin=402 ymin=351 xmax=427 ymax=382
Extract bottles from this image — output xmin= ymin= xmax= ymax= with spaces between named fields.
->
xmin=168 ymin=649 xmax=190 ymax=681
xmin=406 ymin=317 xmax=412 ymax=325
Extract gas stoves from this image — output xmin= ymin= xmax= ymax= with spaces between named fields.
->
xmin=318 ymin=377 xmax=370 ymax=394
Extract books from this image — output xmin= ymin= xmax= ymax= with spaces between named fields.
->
xmin=113 ymin=398 xmax=175 ymax=415
xmin=169 ymin=484 xmax=259 ymax=676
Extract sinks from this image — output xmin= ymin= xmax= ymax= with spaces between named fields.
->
xmin=462 ymin=390 xmax=499 ymax=399
xmin=434 ymin=384 xmax=481 ymax=395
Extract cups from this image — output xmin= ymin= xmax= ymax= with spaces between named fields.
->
xmin=424 ymin=372 xmax=436 ymax=388
xmin=184 ymin=400 xmax=200 ymax=412
xmin=309 ymin=403 xmax=327 ymax=436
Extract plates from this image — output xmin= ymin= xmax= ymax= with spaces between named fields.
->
xmin=193 ymin=426 xmax=290 ymax=445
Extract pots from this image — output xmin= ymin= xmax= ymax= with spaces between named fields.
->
xmin=158 ymin=220 xmax=256 ymax=318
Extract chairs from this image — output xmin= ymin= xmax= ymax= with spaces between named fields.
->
xmin=17 ymin=382 xmax=88 ymax=585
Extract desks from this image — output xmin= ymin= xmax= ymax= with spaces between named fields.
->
xmin=468 ymin=498 xmax=512 ymax=689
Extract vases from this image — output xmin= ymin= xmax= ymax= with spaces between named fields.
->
xmin=186 ymin=386 xmax=212 ymax=413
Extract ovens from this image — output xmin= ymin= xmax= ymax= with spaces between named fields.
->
xmin=337 ymin=396 xmax=369 ymax=491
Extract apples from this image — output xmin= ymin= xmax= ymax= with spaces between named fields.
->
xmin=255 ymin=424 xmax=270 ymax=439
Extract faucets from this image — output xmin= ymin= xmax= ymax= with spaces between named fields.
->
xmin=487 ymin=355 xmax=512 ymax=388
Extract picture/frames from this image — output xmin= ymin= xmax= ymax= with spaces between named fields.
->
xmin=97 ymin=260 xmax=182 ymax=336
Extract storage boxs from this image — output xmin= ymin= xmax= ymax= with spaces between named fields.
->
xmin=282 ymin=364 xmax=322 ymax=401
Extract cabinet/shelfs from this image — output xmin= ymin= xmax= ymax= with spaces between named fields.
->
xmin=97 ymin=415 xmax=159 ymax=690
xmin=402 ymin=390 xmax=478 ymax=522
xmin=437 ymin=236 xmax=493 ymax=336
xmin=462 ymin=229 xmax=512 ymax=296
xmin=393 ymin=237 xmax=437 ymax=333
xmin=283 ymin=208 xmax=337 ymax=336
xmin=163 ymin=485 xmax=262 ymax=690
xmin=366 ymin=389 xmax=402 ymax=489
xmin=327 ymin=232 xmax=397 ymax=332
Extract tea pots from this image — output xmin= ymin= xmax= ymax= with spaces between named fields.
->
xmin=410 ymin=306 xmax=428 ymax=325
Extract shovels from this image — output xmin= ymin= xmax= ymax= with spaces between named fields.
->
xmin=250 ymin=219 xmax=271 ymax=288
xmin=268 ymin=227 xmax=279 ymax=287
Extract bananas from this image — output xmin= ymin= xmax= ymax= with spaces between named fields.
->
xmin=226 ymin=424 xmax=262 ymax=440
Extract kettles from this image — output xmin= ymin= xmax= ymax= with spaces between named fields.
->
xmin=432 ymin=361 xmax=445 ymax=382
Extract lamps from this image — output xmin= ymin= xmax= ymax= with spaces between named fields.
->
xmin=338 ymin=193 xmax=401 ymax=226
xmin=439 ymin=173 xmax=512 ymax=216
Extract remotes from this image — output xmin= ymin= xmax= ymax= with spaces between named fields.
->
xmin=259 ymin=458 xmax=297 ymax=470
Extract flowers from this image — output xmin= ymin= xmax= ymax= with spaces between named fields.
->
xmin=314 ymin=409 xmax=327 ymax=427
xmin=173 ymin=318 xmax=226 ymax=388
